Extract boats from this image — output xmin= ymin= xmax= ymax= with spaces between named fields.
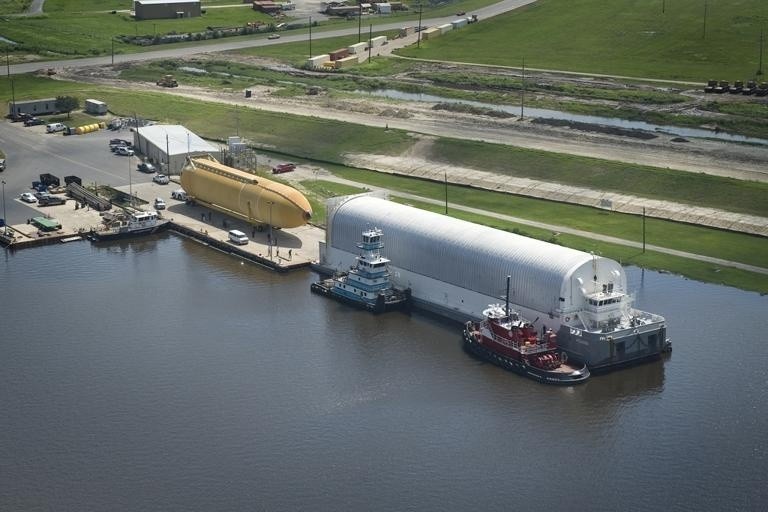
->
xmin=462 ymin=275 xmax=591 ymax=386
xmin=84 ymin=209 xmax=170 ymax=246
xmin=311 ymin=221 xmax=413 ymax=315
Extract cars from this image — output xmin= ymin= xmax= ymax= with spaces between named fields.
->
xmin=267 ymin=33 xmax=280 ymax=39
xmin=137 ymin=162 xmax=155 ymax=173
xmin=15 ymin=113 xmax=33 ymax=122
xmin=35 ymin=192 xmax=47 ymax=199
xmin=20 ymin=193 xmax=37 ymax=203
xmin=456 ymin=10 xmax=465 ymax=16
xmin=171 ymin=189 xmax=187 ymax=201
xmin=25 ymin=117 xmax=45 ymax=126
xmin=110 ymin=139 xmax=135 ymax=156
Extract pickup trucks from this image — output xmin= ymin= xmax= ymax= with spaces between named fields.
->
xmin=153 ymin=174 xmax=169 ymax=185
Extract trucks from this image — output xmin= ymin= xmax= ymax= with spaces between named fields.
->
xmin=38 ymin=195 xmax=63 ymax=206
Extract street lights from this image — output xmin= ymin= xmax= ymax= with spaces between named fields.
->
xmin=2 ymin=180 xmax=7 ymax=235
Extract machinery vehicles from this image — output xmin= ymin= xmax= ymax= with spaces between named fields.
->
xmin=156 ymin=74 xmax=178 ymax=88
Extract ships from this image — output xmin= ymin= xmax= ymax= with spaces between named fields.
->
xmin=309 ymin=187 xmax=674 ymax=376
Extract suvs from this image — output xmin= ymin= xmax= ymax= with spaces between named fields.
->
xmin=273 ymin=162 xmax=296 ymax=173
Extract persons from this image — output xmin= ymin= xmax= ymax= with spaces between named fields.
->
xmin=201 ymin=210 xmax=292 ymax=259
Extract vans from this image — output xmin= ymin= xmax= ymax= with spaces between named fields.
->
xmin=47 ymin=67 xmax=57 ymax=75
xmin=228 ymin=229 xmax=248 ymax=245
xmin=47 ymin=123 xmax=65 ymax=132
xmin=155 ymin=197 xmax=165 ymax=210
